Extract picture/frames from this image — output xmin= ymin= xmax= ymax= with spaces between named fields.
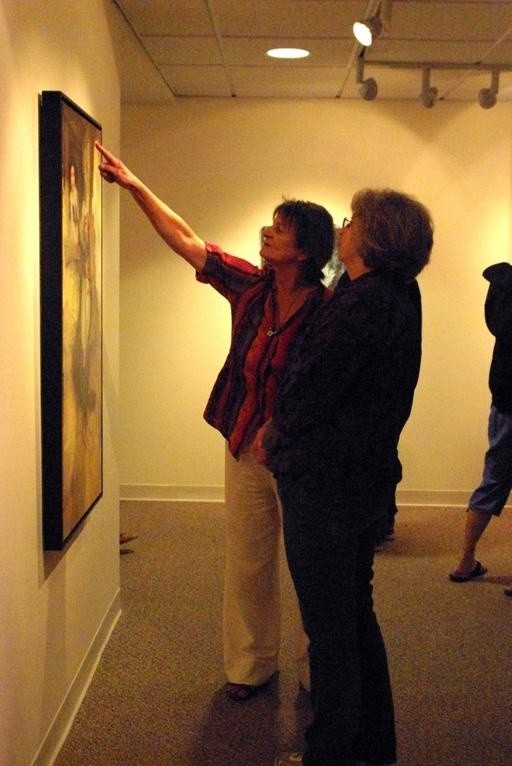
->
xmin=39 ymin=87 xmax=105 ymax=551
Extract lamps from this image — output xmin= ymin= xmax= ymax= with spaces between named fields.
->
xmin=478 ymin=69 xmax=500 ymax=110
xmin=350 ymin=0 xmax=387 ymax=48
xmin=354 ymin=59 xmax=377 ymax=102
xmin=419 ymin=65 xmax=439 ymax=109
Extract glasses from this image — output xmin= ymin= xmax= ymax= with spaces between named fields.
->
xmin=343 ymin=216 xmax=368 ymax=233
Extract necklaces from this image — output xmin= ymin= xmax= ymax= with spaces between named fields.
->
xmin=265 ymin=282 xmax=306 ymax=336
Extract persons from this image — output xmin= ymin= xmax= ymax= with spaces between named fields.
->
xmin=250 ymin=186 xmax=436 ymax=764
xmin=68 ymin=162 xmax=80 ymax=250
xmin=373 ymin=455 xmax=405 ymax=554
xmin=94 ymin=140 xmax=337 ymax=703
xmin=447 ymin=261 xmax=511 ymax=597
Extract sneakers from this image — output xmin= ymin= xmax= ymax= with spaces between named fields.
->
xmin=275 ymin=751 xmax=304 ymax=766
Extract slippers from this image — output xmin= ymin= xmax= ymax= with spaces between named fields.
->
xmin=450 ymin=560 xmax=488 ymax=582
xmin=227 ymin=670 xmax=278 ymax=700
